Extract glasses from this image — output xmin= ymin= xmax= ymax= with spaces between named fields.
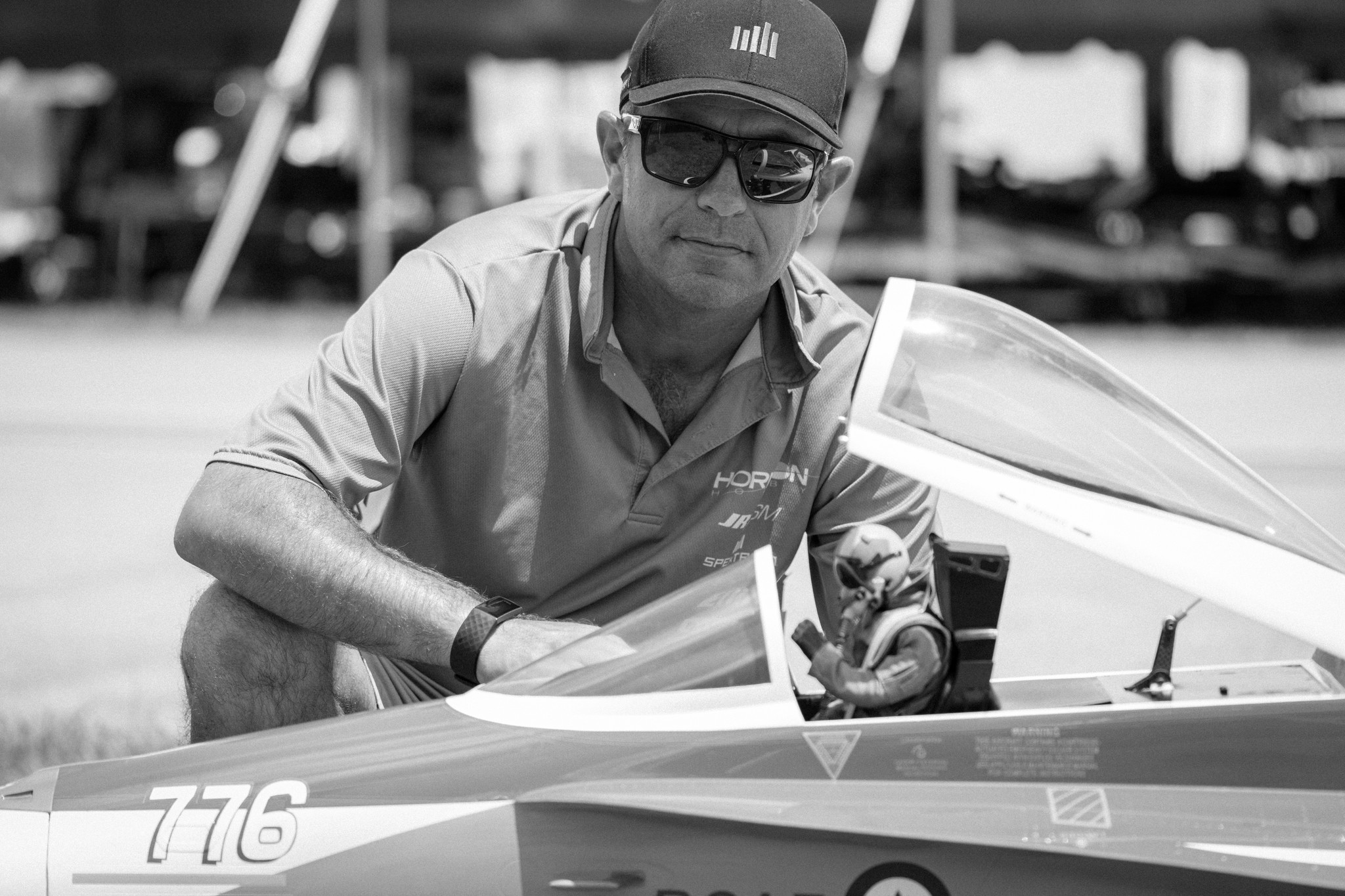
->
xmin=617 ymin=113 xmax=828 ymax=204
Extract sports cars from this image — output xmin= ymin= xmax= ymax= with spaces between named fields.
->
xmin=0 ymin=277 xmax=1345 ymax=896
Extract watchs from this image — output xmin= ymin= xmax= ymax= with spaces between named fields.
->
xmin=450 ymin=596 xmax=523 ymax=690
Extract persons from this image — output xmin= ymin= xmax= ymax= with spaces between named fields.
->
xmin=174 ymin=1 xmax=938 ymax=743
xmin=790 ymin=524 xmax=948 ymax=722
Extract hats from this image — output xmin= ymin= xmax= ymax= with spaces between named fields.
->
xmin=619 ymin=0 xmax=849 ymax=150
